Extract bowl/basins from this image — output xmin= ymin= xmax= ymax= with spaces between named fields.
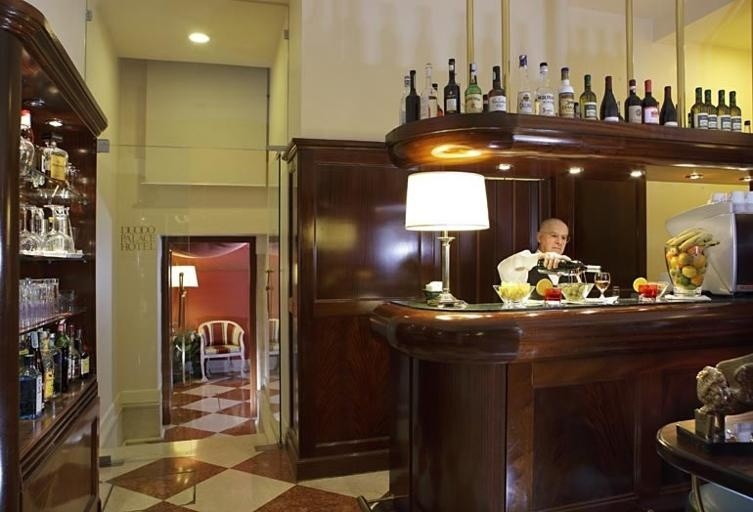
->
xmin=636 ymin=283 xmax=670 ymax=301
xmin=419 ymin=288 xmax=454 ymax=302
xmin=492 ymin=285 xmax=535 ymax=305
xmin=558 ymin=283 xmax=595 ymax=302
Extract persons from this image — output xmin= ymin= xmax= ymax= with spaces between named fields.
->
xmin=497 ymin=218 xmax=583 ymax=301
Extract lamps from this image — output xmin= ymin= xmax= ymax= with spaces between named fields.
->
xmin=402 ymin=169 xmax=491 ymax=309
xmin=170 ymin=264 xmax=199 ymax=331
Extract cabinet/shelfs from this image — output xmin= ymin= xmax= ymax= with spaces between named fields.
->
xmin=1 ymin=1 xmax=109 ymax=510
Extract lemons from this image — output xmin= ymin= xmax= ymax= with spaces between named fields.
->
xmin=664 ymin=249 xmax=706 ymax=289
xmin=633 ymin=277 xmax=647 ymax=292
xmin=536 ymin=279 xmax=552 ymax=295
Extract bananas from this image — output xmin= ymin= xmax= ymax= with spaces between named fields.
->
xmin=663 ymin=227 xmax=718 ymax=247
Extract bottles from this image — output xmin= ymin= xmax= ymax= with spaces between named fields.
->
xmin=18 ymin=136 xmax=35 ymax=176
xmin=534 ymin=62 xmax=555 ymax=117
xmin=398 ymin=62 xmax=442 ymax=125
xmin=463 ymin=63 xmax=483 ymax=113
xmin=35 ymin=141 xmax=80 ymax=182
xmin=559 ymin=67 xmax=624 ymax=122
xmin=536 ymin=258 xmax=601 ymax=277
xmin=486 ymin=65 xmax=506 ymax=112
xmin=690 ymin=87 xmax=750 ymax=134
xmin=516 ymin=54 xmax=532 ymax=113
xmin=443 ymin=59 xmax=460 ymax=115
xmin=20 ymin=109 xmax=33 ymax=142
xmin=623 ymin=80 xmax=677 ymax=127
xmin=18 ymin=322 xmax=90 ymax=420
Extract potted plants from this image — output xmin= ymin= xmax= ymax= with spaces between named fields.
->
xmin=170 ymin=328 xmax=201 ymax=378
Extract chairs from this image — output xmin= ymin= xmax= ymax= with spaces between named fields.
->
xmin=683 ymin=355 xmax=751 ymax=511
xmin=195 ymin=315 xmax=247 ymax=384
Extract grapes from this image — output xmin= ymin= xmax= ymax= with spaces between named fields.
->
xmin=564 ymin=285 xmax=586 ymax=300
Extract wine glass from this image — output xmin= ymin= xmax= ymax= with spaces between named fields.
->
xmin=20 ymin=203 xmax=73 ymax=254
xmin=594 ymin=272 xmax=610 ymax=302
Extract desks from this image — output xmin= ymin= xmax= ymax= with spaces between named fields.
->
xmin=653 ymin=411 xmax=753 ymax=512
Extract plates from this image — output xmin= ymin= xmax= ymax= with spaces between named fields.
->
xmin=664 ymin=294 xmax=711 ymax=303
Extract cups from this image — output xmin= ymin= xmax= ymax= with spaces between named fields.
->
xmin=16 ymin=277 xmax=74 ymax=328
xmin=664 ymin=245 xmax=709 ymax=298
xmin=542 ymin=287 xmax=562 ymax=305
xmin=708 ymin=191 xmax=752 ymax=205
